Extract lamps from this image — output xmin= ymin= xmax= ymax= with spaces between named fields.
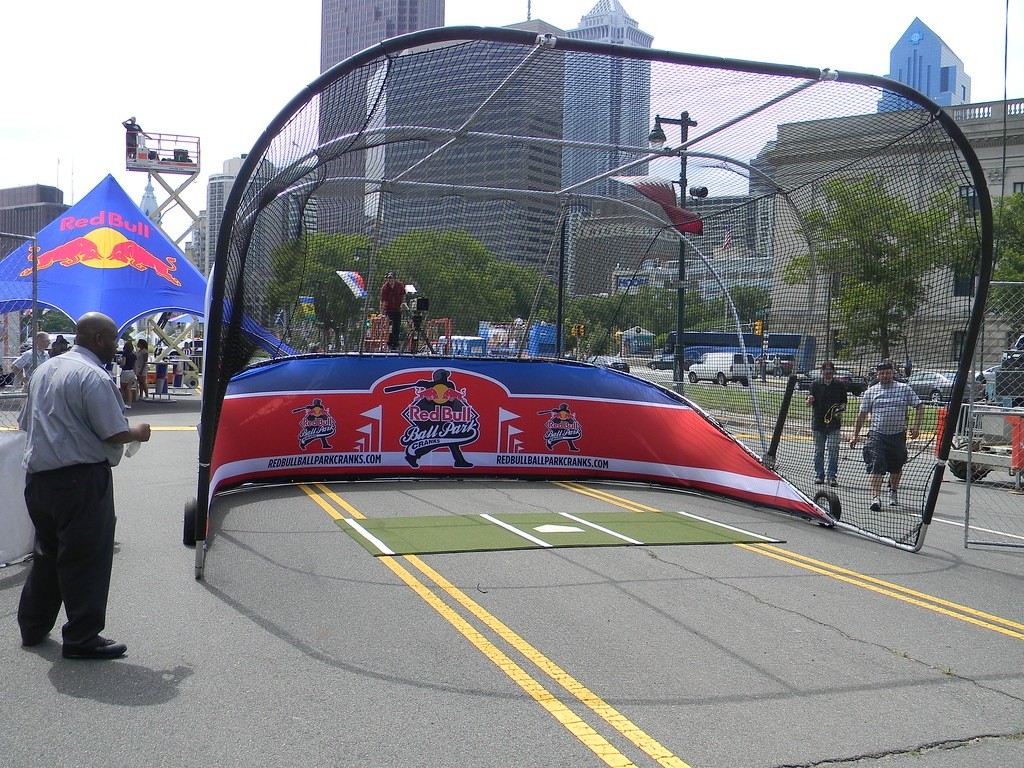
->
xmin=689 ymin=186 xmax=708 ymax=198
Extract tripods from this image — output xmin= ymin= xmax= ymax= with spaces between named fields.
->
xmin=398 ymin=306 xmax=436 ymax=355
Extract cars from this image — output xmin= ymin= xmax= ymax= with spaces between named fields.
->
xmin=794 ymin=365 xmax=868 ymax=396
xmin=647 ymin=353 xmax=692 ymax=371
xmin=902 ymin=369 xmax=984 ymax=405
xmin=974 ymin=363 xmax=1002 ymax=384
xmin=588 ymin=354 xmax=631 ymax=373
xmin=20 ymin=333 xmax=77 ymax=353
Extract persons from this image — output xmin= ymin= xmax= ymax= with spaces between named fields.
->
xmin=134 ymin=339 xmax=149 ymax=399
xmin=10 ymin=331 xmax=51 ymax=384
xmin=904 ymin=360 xmax=912 ymax=378
xmin=49 ymin=334 xmax=70 ymax=357
xmin=515 ymin=315 xmax=523 ymax=326
xmin=541 ymin=319 xmax=548 ymax=326
xmin=381 ymin=270 xmax=410 ymax=349
xmin=848 ymin=359 xmax=925 ymax=511
xmin=312 ymin=346 xmax=320 ymax=353
xmin=772 ymin=354 xmax=783 ymax=378
xmin=17 ymin=311 xmax=151 ymax=659
xmin=805 ymin=360 xmax=868 ymax=486
xmin=122 ymin=116 xmax=151 ymax=162
xmin=976 ymin=373 xmax=986 ymax=384
xmin=119 ymin=340 xmax=138 ymax=408
xmin=898 ymin=362 xmax=903 ymax=368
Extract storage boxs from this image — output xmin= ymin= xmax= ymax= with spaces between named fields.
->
xmin=997 ymin=351 xmax=1024 ymax=397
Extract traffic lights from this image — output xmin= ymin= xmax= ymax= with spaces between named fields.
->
xmin=614 ymin=332 xmax=622 ymax=343
xmin=580 ymin=324 xmax=586 ymax=336
xmin=571 ymin=328 xmax=576 ymax=336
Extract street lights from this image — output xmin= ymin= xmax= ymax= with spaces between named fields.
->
xmin=647 ymin=109 xmax=698 ymax=398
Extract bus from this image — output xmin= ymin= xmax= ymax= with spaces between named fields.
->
xmin=666 ymin=330 xmax=818 ymax=372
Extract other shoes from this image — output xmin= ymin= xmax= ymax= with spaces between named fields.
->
xmin=124 ymin=405 xmax=131 ymax=409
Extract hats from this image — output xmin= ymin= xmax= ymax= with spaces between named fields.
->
xmin=60 ymin=339 xmax=69 ymax=344
xmin=822 ymin=361 xmax=834 ymax=373
xmin=387 ymin=272 xmax=395 ymax=278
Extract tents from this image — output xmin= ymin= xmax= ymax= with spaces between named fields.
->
xmin=0 ymin=172 xmax=296 ymax=389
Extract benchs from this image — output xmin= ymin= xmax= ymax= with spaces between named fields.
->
xmin=147 ymin=372 xmax=175 ymax=384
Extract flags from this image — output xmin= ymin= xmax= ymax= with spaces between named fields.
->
xmin=723 ymin=224 xmax=731 ymax=250
xmin=610 ymin=174 xmax=703 ymax=236
xmin=336 ymin=270 xmax=366 ymax=299
xmin=299 ymin=295 xmax=316 ymax=320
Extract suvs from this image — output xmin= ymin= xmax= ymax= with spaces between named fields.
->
xmin=756 ymin=352 xmax=795 ymax=376
xmin=164 ymin=337 xmax=204 ymax=367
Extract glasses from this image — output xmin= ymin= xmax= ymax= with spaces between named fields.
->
xmin=878 ymin=363 xmax=889 ymax=367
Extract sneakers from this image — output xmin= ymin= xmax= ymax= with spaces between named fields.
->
xmin=888 ymin=488 xmax=897 ymax=505
xmin=869 ymin=496 xmax=881 ymax=511
xmin=815 ymin=476 xmax=824 ymax=484
xmin=62 ymin=639 xmax=128 ymax=659
xmin=828 ymin=479 xmax=838 ymax=487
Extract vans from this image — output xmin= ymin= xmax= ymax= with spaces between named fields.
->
xmin=688 ymin=352 xmax=758 ymax=386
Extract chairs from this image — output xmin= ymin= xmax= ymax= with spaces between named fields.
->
xmin=365 ymin=315 xmax=450 ymax=354
